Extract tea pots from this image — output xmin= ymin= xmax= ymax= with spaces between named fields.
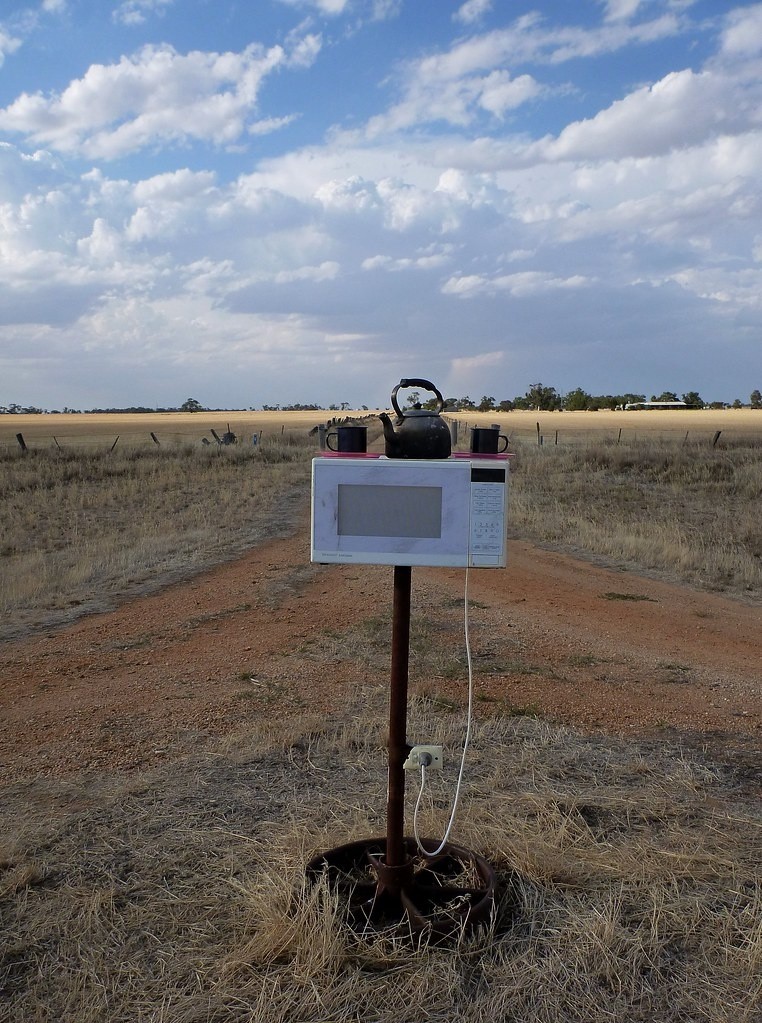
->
xmin=377 ymin=377 xmax=452 ymax=458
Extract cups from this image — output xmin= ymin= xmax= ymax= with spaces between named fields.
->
xmin=326 ymin=426 xmax=369 ymax=452
xmin=470 ymin=427 xmax=509 ymax=454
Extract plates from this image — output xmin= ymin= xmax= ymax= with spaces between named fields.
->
xmin=453 ymin=452 xmax=516 ymax=459
xmin=315 ymin=451 xmax=384 ymax=458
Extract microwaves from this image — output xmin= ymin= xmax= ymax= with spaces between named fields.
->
xmin=311 ymin=457 xmax=510 ymax=570
